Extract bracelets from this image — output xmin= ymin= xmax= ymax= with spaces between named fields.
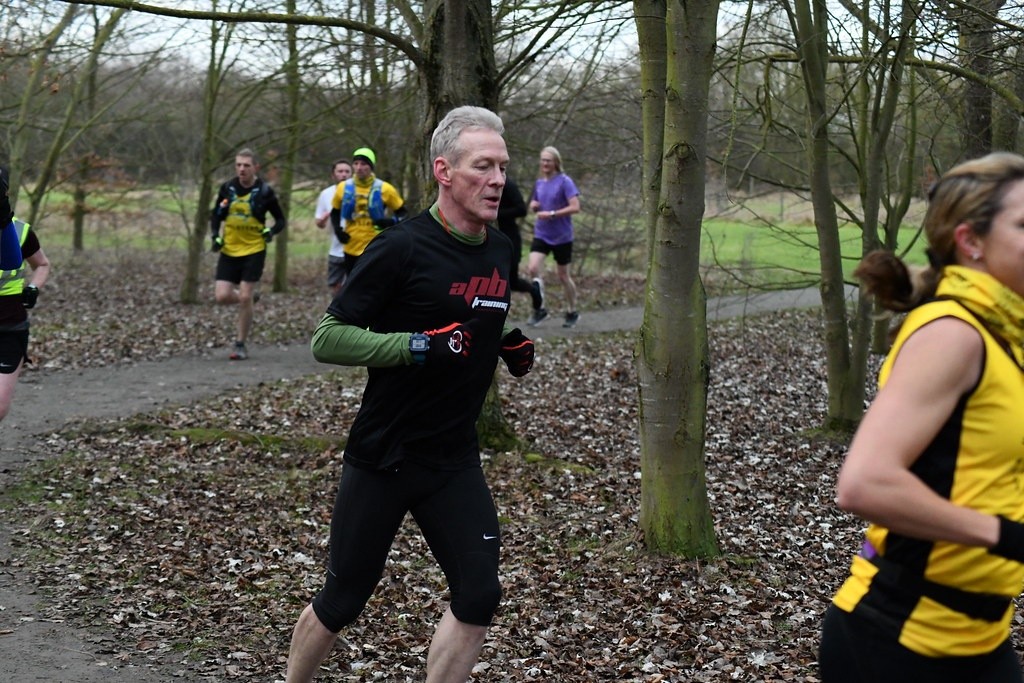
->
xmin=985 ymin=511 xmax=1024 ymax=562
xmin=551 ymin=210 xmax=555 ymax=219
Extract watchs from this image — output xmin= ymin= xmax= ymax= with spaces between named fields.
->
xmin=409 ymin=333 xmax=430 ymax=353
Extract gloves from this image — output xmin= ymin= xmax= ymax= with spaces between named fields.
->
xmin=262 ymin=227 xmax=272 ymax=242
xmin=499 ymin=328 xmax=534 ymax=377
xmin=212 ymin=237 xmax=223 ymax=251
xmin=423 ymin=320 xmax=480 ymax=363
xmin=988 ymin=514 xmax=1024 ymax=566
xmin=335 ymin=227 xmax=350 ymax=244
xmin=372 ymin=218 xmax=395 ymax=230
xmin=22 ymin=285 xmax=39 ymax=309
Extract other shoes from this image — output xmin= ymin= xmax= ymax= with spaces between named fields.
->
xmin=563 ymin=311 xmax=580 ymax=328
xmin=230 ymin=347 xmax=248 ymax=360
xmin=531 ymin=278 xmax=544 ymax=310
xmin=526 ymin=309 xmax=550 ymax=326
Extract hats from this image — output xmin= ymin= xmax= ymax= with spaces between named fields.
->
xmin=352 ymin=147 xmax=376 ymax=168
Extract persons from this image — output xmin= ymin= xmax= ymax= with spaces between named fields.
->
xmin=528 ymin=146 xmax=581 ymax=329
xmin=286 ymin=104 xmax=535 ymax=683
xmin=0 ymin=159 xmax=22 ymax=271
xmin=213 ymin=148 xmax=286 ymax=360
xmin=496 ymin=176 xmax=545 ymax=310
xmin=0 ymin=215 xmax=51 ymax=422
xmin=330 ymin=147 xmax=408 ymax=279
xmin=314 ymin=157 xmax=354 ymax=300
xmin=817 ymin=151 xmax=1024 ymax=683
